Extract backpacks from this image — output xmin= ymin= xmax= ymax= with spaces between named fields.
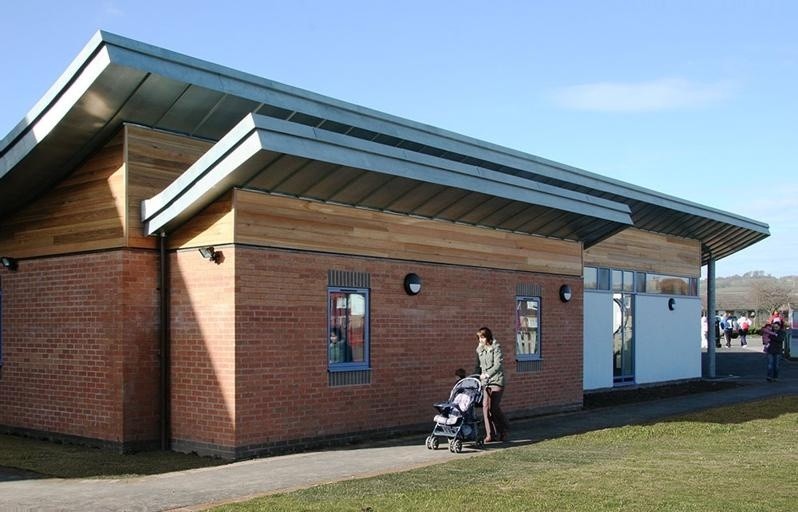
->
xmin=739 ymin=321 xmax=749 ymax=330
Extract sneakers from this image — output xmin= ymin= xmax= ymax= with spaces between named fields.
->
xmin=724 ymin=343 xmax=749 ymax=349
xmin=483 ymin=431 xmax=507 ymax=442
xmin=766 ymin=376 xmax=779 ymax=384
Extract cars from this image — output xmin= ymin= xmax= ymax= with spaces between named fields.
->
xmin=714 ymin=314 xmax=737 ymax=339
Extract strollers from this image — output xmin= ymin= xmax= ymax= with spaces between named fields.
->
xmin=421 ymin=372 xmax=489 ymax=455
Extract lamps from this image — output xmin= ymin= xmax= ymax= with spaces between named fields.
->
xmin=198 ymin=245 xmax=222 ymax=264
xmin=404 ymin=272 xmax=422 ymax=296
xmin=559 ymin=284 xmax=573 ymax=303
xmin=667 ymin=297 xmax=677 ymax=310
xmin=0 ymin=256 xmax=19 ymax=270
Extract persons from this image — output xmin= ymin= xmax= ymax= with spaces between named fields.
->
xmin=455 ymin=367 xmax=466 ymax=393
xmin=761 ymin=321 xmax=784 ymax=381
xmin=737 ymin=311 xmax=752 ymax=347
xmin=762 ymin=324 xmax=777 ymax=351
xmin=331 ymin=329 xmax=353 ymax=363
xmin=718 ymin=311 xmax=735 ymax=349
xmin=768 ymin=308 xmax=784 ymax=323
xmin=475 ymin=325 xmax=509 ymax=444
